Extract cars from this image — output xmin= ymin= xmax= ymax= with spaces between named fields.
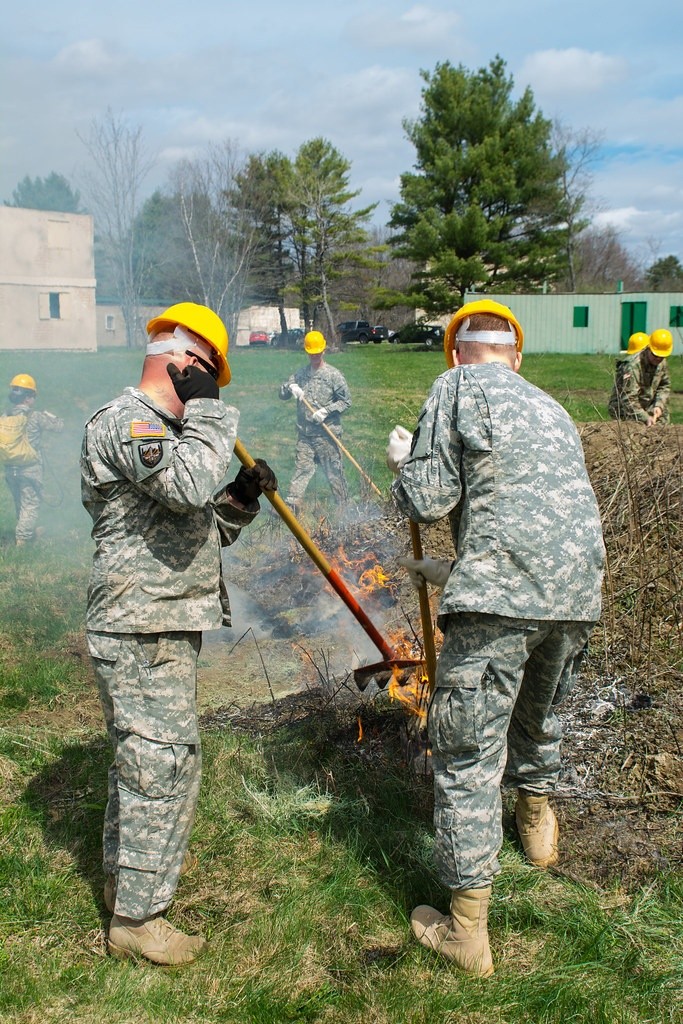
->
xmin=249 ymin=328 xmax=304 ymax=348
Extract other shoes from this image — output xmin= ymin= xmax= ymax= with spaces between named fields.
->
xmin=335 ymin=499 xmax=355 ymax=517
xmin=36 ymin=527 xmax=45 ymax=538
xmin=16 ymin=539 xmax=27 ymax=546
xmin=268 ymin=507 xmax=296 ymax=518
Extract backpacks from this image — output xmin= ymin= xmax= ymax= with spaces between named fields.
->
xmin=0 ymin=409 xmax=38 ymax=467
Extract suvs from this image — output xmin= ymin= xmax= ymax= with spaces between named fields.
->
xmin=388 ymin=324 xmax=445 ymax=346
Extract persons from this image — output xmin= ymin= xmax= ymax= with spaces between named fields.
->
xmin=609 ymin=329 xmax=674 ymax=427
xmin=268 ymin=330 xmax=352 ymax=520
xmin=0 ymin=374 xmax=64 ymax=548
xmin=80 ymin=302 xmax=278 ymax=964
xmin=388 ymin=299 xmax=605 ymax=979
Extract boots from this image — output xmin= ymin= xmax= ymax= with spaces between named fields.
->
xmin=103 ymin=852 xmax=199 ymax=912
xmin=108 ymin=915 xmax=208 ymax=968
xmin=515 ymin=788 xmax=559 ymax=868
xmin=409 ymin=885 xmax=495 ymax=979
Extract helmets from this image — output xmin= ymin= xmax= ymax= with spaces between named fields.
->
xmin=304 ymin=331 xmax=326 ymax=354
xmin=146 ymin=302 xmax=231 ymax=389
xmin=10 ymin=374 xmax=36 ymax=393
xmin=627 ymin=332 xmax=649 ymax=355
xmin=443 ymin=299 xmax=523 ymax=369
xmin=648 ymin=328 xmax=673 ymax=357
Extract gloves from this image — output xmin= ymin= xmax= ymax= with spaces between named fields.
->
xmin=313 ymin=408 xmax=329 ymax=425
xmin=289 ymin=384 xmax=305 ymax=401
xmin=44 ymin=411 xmax=56 ymax=419
xmin=167 ymin=363 xmax=219 ymax=405
xmin=386 ymin=425 xmax=413 ymax=475
xmin=395 ymin=556 xmax=455 ymax=589
xmin=227 ymin=458 xmax=278 ymax=507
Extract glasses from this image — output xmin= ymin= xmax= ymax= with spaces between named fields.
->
xmin=186 ymin=350 xmax=221 ymax=381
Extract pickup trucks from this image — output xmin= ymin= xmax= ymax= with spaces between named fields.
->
xmin=336 ymin=320 xmax=388 ymax=344
